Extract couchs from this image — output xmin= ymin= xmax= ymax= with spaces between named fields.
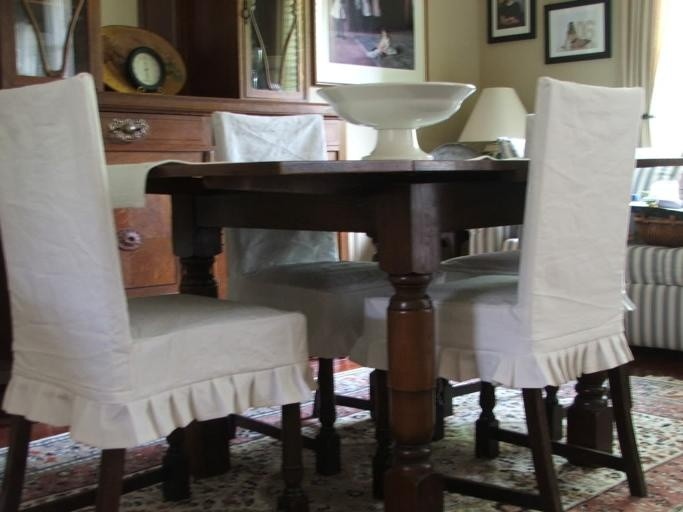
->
xmin=432 ymin=139 xmax=682 ymax=352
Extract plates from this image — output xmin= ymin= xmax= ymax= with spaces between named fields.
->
xmin=99 ymin=24 xmax=187 ymax=95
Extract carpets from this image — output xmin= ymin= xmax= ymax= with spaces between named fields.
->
xmin=1 ymin=367 xmax=681 ymax=512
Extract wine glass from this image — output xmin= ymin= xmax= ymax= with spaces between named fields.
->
xmin=316 ymin=82 xmax=477 ymax=162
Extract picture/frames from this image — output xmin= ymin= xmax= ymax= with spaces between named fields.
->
xmin=310 ymin=0 xmax=429 ymax=88
xmin=488 ymin=0 xmax=611 ymax=64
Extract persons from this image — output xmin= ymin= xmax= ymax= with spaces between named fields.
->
xmin=561 ymin=22 xmax=593 ymax=51
xmin=367 ymin=28 xmax=397 ymax=62
xmin=329 ymin=1 xmax=349 ymax=40
xmin=498 ymin=0 xmax=523 ymax=29
